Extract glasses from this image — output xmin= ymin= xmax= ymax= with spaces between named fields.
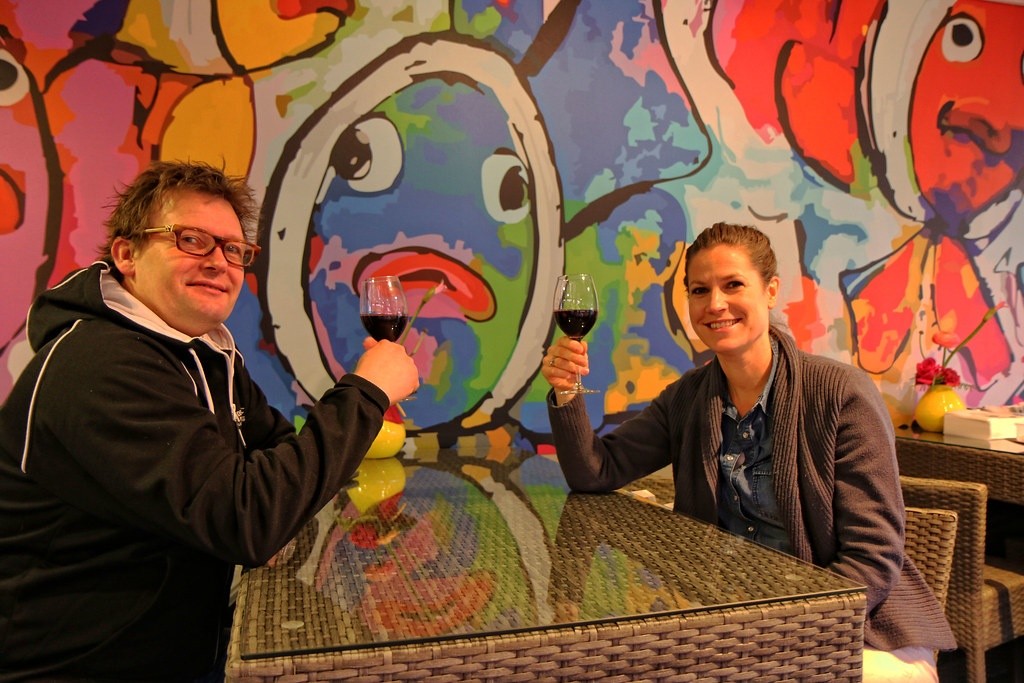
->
xmin=142 ymin=223 xmax=262 ymax=268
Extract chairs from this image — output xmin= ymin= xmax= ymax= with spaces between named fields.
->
xmin=898 ymin=475 xmax=1024 ymax=683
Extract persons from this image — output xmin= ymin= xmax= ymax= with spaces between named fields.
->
xmin=0 ymin=160 xmax=420 ymax=683
xmin=541 ymin=222 xmax=956 ymax=683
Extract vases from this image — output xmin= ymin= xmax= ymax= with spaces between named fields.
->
xmin=913 ymin=385 xmax=966 ymax=433
xmin=362 ymin=405 xmax=406 ymax=459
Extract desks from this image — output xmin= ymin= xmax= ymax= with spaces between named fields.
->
xmin=894 ymin=415 xmax=1024 ymax=503
xmin=227 ymin=446 xmax=869 ymax=683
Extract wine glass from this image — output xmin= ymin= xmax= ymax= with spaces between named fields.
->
xmin=552 ymin=273 xmax=600 ymax=395
xmin=360 ymin=275 xmax=418 ymax=403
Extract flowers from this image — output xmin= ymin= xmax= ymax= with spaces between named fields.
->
xmin=915 ymin=301 xmax=1010 ymax=386
xmin=394 ymin=279 xmax=447 ymax=417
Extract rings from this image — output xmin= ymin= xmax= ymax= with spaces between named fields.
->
xmin=548 ymin=355 xmax=556 ymax=367
